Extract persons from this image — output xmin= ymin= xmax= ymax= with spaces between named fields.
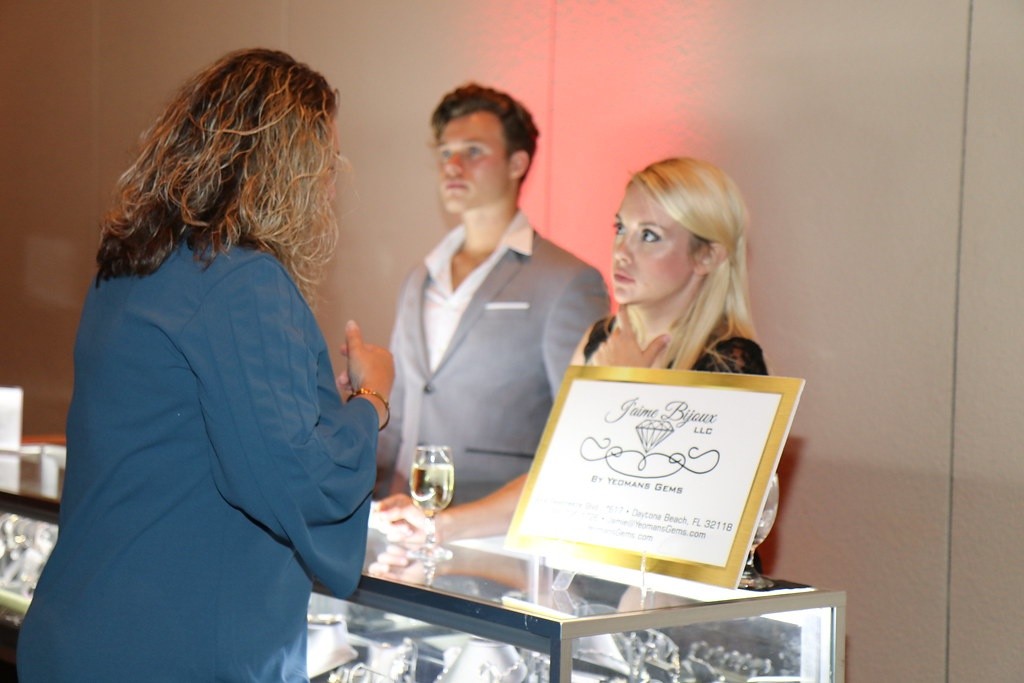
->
xmin=12 ymin=47 xmax=395 ymax=683
xmin=372 ymin=83 xmax=610 ymax=516
xmin=370 ymin=539 xmax=701 ymax=615
xmin=380 ymin=159 xmax=768 ymax=577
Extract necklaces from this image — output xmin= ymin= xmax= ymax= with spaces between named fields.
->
xmin=433 ymin=654 xmax=525 ymax=683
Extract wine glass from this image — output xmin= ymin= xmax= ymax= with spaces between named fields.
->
xmin=407 ymin=445 xmax=454 ymax=560
xmin=736 ymin=474 xmax=779 ymax=590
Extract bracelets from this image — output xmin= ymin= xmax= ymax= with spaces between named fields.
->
xmin=348 ymin=662 xmax=373 ymax=683
xmin=346 ymin=388 xmax=391 ymax=431
